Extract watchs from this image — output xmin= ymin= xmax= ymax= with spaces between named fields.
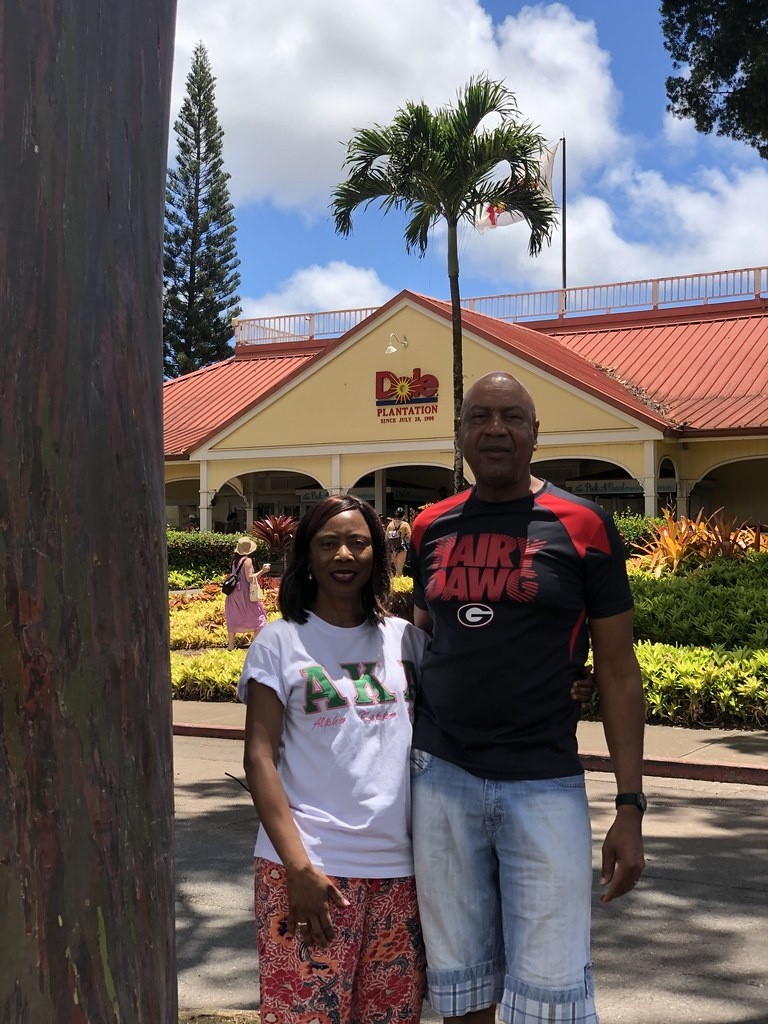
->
xmin=615 ymin=793 xmax=648 ymax=810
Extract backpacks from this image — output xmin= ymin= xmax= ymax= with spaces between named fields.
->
xmin=388 ymin=523 xmax=407 ymax=551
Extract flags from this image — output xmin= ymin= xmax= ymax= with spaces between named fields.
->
xmin=478 ymin=145 xmax=558 ymax=233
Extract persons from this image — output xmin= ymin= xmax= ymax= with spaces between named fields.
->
xmin=223 ymin=537 xmax=271 ymax=650
xmin=408 ymin=372 xmax=647 ymax=1024
xmin=237 ymin=494 xmax=430 ymax=1023
xmin=185 ymin=514 xmax=195 ymax=526
xmin=380 ymin=508 xmax=409 ymax=577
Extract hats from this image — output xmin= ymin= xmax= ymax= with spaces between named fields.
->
xmin=234 ymin=537 xmax=257 ymax=556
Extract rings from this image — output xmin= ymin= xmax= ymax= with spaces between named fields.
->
xmin=298 ymin=922 xmax=308 ymax=926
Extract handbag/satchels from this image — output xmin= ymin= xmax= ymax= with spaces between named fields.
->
xmin=249 ymin=575 xmax=262 ymax=601
xmin=222 ymin=574 xmax=239 ymax=596
